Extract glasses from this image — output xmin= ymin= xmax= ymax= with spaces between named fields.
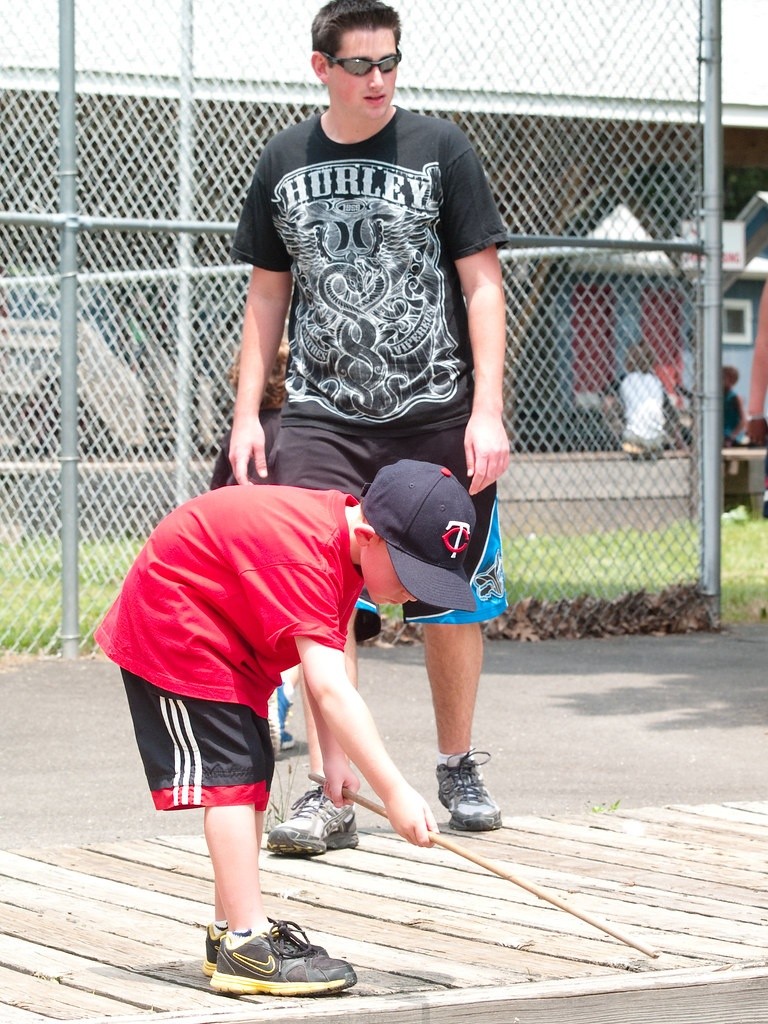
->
xmin=320 ymin=46 xmax=402 ymax=77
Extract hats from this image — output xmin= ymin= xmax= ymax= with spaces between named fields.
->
xmin=360 ymin=458 xmax=477 ymax=613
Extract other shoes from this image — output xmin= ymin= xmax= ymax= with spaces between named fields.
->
xmin=280 ymin=732 xmax=294 ymax=748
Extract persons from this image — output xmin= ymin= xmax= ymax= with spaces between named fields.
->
xmin=228 ymin=0 xmax=511 ymax=859
xmin=210 ymin=344 xmax=297 ymax=755
xmin=601 ymin=342 xmax=691 ymax=456
xmin=674 ymin=367 xmax=747 ymax=450
xmin=747 ymin=282 xmax=768 ymax=618
xmin=93 ymin=459 xmax=475 ymax=993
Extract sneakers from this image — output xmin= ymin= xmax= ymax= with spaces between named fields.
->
xmin=435 ymin=748 xmax=502 ymax=831
xmin=203 ymin=924 xmax=229 ymax=977
xmin=209 ymin=920 xmax=357 ymax=996
xmin=267 ymin=785 xmax=359 ymax=855
